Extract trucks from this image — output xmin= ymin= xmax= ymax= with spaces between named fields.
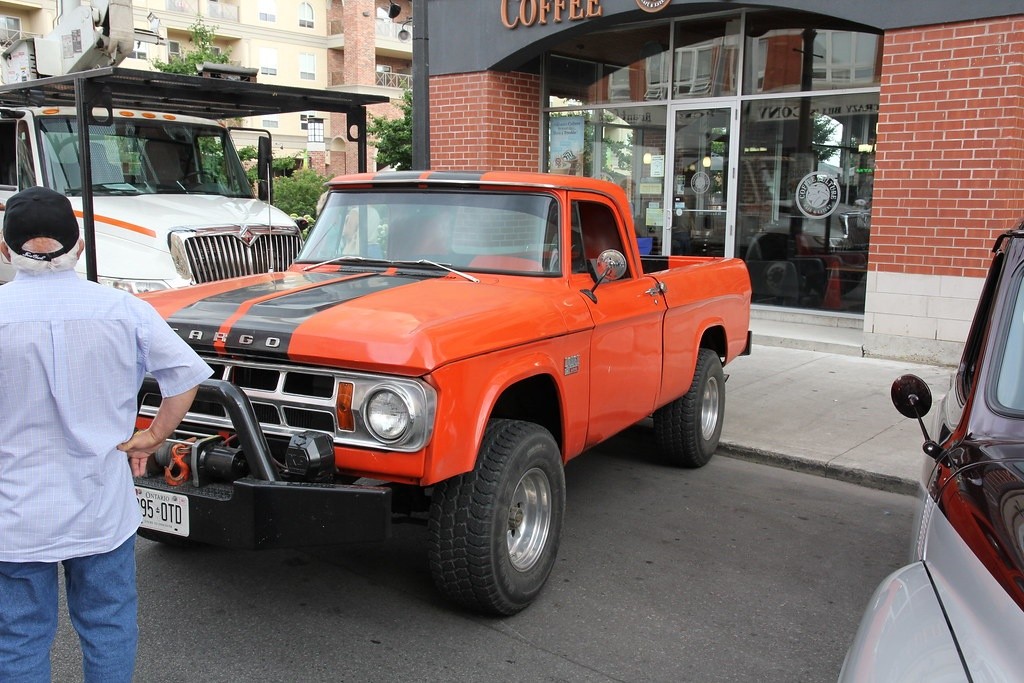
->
xmin=0 ymin=0 xmax=390 ymax=294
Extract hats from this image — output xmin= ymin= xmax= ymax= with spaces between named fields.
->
xmin=2 ymin=186 xmax=79 ymax=262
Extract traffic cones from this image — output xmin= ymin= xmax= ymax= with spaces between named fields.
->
xmin=821 ymin=261 xmax=843 ymax=310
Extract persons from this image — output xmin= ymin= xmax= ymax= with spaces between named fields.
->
xmin=0 ymin=185 xmax=215 ymax=683
xmin=672 ymin=195 xmax=696 ymax=256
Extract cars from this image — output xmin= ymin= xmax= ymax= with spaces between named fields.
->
xmin=836 ymin=215 xmax=1024 ymax=683
xmin=671 ymin=156 xmax=871 ymax=305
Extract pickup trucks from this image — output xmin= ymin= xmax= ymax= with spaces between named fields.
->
xmin=134 ymin=170 xmax=752 ymax=619
